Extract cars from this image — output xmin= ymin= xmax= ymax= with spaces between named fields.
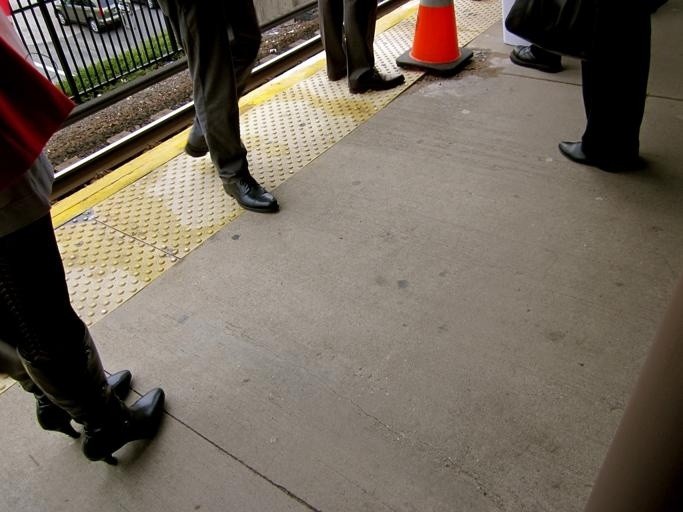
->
xmin=53 ymin=0 xmax=158 ymax=33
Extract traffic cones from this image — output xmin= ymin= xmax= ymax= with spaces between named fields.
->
xmin=395 ymin=0 xmax=473 ymax=77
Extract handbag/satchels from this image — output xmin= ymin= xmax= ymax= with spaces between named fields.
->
xmin=504 ymin=0 xmax=586 ymax=59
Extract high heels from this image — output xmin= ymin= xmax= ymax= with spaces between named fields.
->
xmin=0 ymin=338 xmax=134 ymax=439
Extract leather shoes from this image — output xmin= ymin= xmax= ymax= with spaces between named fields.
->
xmin=185 ymin=142 xmax=210 ymax=157
xmin=509 ymin=44 xmax=563 ymax=74
xmin=557 ymin=140 xmax=639 ymax=175
xmin=222 ymin=172 xmax=279 ymax=213
xmin=328 ymin=71 xmax=405 ymax=93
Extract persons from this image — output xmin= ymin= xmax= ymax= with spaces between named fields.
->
xmin=555 ymin=1 xmax=657 ymax=175
xmin=315 ymin=1 xmax=406 ymax=95
xmin=153 ymin=1 xmax=283 ymax=215
xmin=508 ymin=41 xmax=565 ymax=76
xmin=0 ymin=3 xmax=167 ymax=469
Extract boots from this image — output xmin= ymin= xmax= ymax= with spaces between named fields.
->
xmin=15 ymin=320 xmax=167 ymax=466
